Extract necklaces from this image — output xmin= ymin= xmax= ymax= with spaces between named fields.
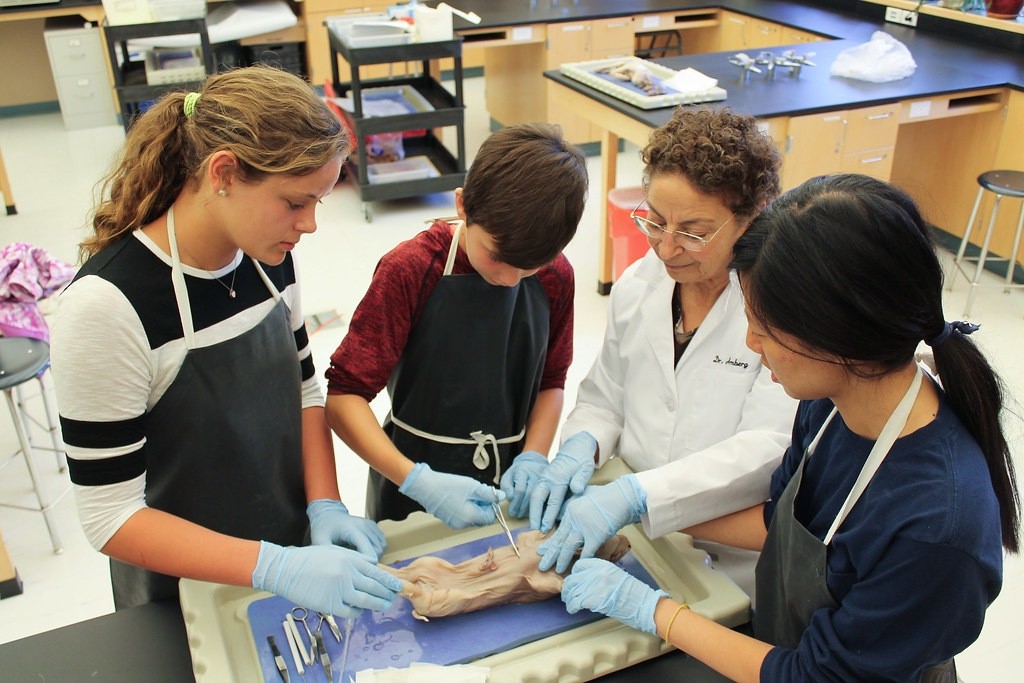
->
xmin=178 ymin=243 xmax=237 ymax=298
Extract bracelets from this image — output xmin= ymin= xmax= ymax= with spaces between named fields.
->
xmin=666 ymin=604 xmax=691 ymax=647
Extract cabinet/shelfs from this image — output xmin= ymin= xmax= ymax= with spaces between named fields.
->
xmin=43 ymin=15 xmax=120 ymax=127
xmin=304 ymin=2 xmax=445 ymax=149
xmin=323 ymin=15 xmax=465 ymax=223
xmin=100 ymin=17 xmax=217 ymax=132
xmin=749 ymin=105 xmax=889 ymax=198
xmin=485 ymin=16 xmax=635 ymax=148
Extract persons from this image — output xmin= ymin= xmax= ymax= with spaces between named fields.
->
xmin=49 ymin=67 xmax=404 ymax=619
xmin=324 ymin=123 xmax=587 ymax=531
xmin=545 ymin=173 xmax=1023 ymax=683
xmin=529 ymin=108 xmax=800 ymax=574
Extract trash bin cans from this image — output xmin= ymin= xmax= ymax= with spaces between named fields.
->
xmin=608 ymin=187 xmax=653 ymax=285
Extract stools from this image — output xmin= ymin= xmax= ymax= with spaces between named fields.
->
xmin=948 ymin=170 xmax=1024 ymax=316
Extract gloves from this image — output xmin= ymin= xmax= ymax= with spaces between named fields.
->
xmin=501 ymin=448 xmax=548 ymax=519
xmin=307 ymin=498 xmax=388 ymax=567
xmin=397 ymin=461 xmax=505 ymax=530
xmin=537 ymin=475 xmax=645 ymax=574
xmin=529 ymin=431 xmax=597 ymax=534
xmin=560 ymin=557 xmax=671 ymax=642
xmin=254 ymin=537 xmax=403 ymax=619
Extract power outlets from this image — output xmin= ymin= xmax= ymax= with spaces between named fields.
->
xmin=885 ymin=7 xmax=918 ymax=28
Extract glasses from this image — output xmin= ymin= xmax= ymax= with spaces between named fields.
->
xmin=631 ymin=193 xmax=743 ymax=252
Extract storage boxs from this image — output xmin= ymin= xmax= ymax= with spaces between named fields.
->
xmin=255 ymin=42 xmax=301 ymax=81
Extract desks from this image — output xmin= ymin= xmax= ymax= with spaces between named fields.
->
xmin=0 ymin=4 xmax=126 ymax=130
xmin=0 ymin=598 xmax=752 ymax=683
xmin=399 ymin=0 xmax=1024 ymax=299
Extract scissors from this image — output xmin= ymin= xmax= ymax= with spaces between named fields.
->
xmin=320 ymin=611 xmax=343 ymax=643
xmin=291 ymin=606 xmax=325 ymax=666
xmin=490 ymin=486 xmax=520 ymax=558
